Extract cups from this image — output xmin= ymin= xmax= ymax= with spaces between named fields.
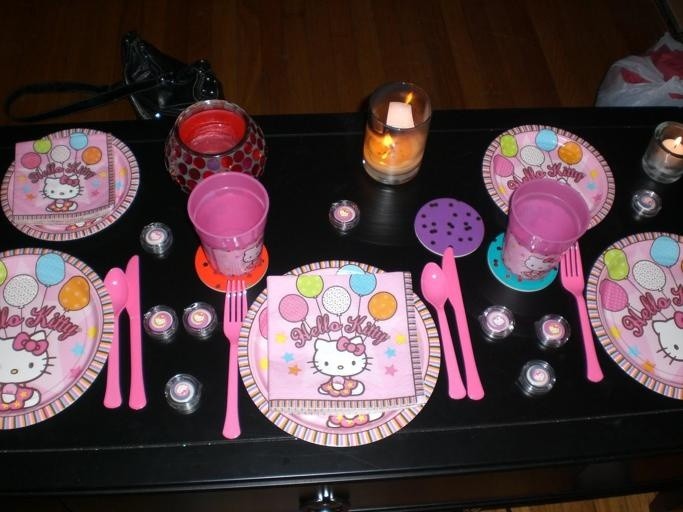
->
xmin=502 ymin=180 xmax=591 ymax=280
xmin=642 ymin=122 xmax=683 ymax=184
xmin=188 ymin=171 xmax=269 ymax=278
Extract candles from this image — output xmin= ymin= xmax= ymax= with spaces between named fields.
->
xmin=653 ymin=137 xmax=683 ymax=170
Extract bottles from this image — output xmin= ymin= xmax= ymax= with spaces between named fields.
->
xmin=163 ymin=99 xmax=268 ymax=197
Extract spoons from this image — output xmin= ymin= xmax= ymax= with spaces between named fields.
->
xmin=102 ymin=267 xmax=127 ymax=408
xmin=421 ymin=263 xmax=467 ymax=401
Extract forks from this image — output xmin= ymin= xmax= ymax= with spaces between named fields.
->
xmin=222 ymin=278 xmax=248 ymax=440
xmin=560 ymin=241 xmax=604 ymax=384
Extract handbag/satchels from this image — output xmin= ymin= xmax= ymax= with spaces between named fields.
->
xmin=121 ymin=31 xmax=225 ymax=120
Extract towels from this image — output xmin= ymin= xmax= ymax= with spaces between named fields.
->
xmin=12 ymin=133 xmax=116 ymax=224
xmin=265 ymin=270 xmax=425 ymax=415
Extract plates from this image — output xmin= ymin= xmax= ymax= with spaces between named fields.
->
xmin=587 ymin=232 xmax=683 ymax=401
xmin=1 ymin=128 xmax=140 ymax=241
xmin=237 ymin=261 xmax=442 ymax=447
xmin=0 ymin=248 xmax=114 ymax=432
xmin=481 ymin=124 xmax=616 ymax=232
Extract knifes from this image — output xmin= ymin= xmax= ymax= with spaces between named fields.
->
xmin=124 ymin=255 xmax=149 ymax=408
xmin=442 ymin=247 xmax=486 ymax=400
